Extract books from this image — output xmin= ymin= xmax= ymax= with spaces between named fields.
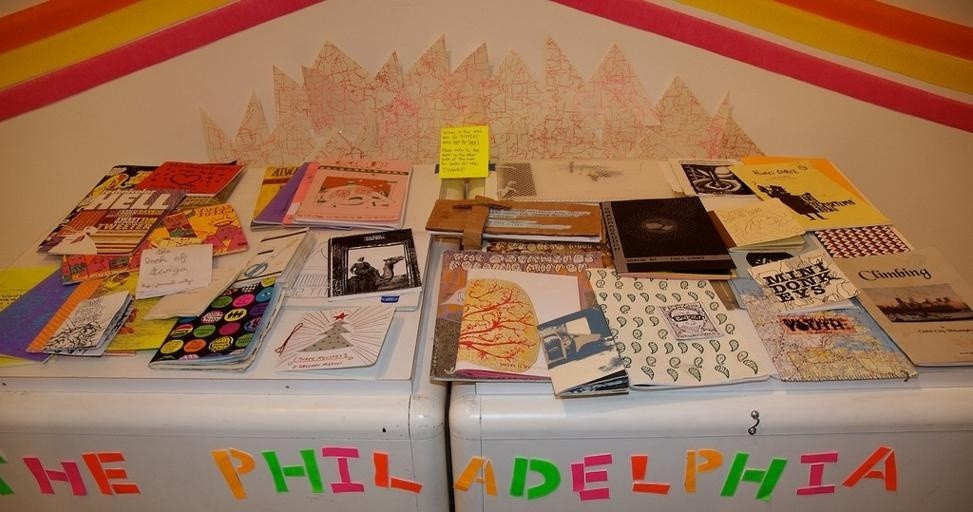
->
xmin=0 ymin=157 xmax=422 ymax=381
xmin=426 ymin=156 xmax=971 ymax=396
